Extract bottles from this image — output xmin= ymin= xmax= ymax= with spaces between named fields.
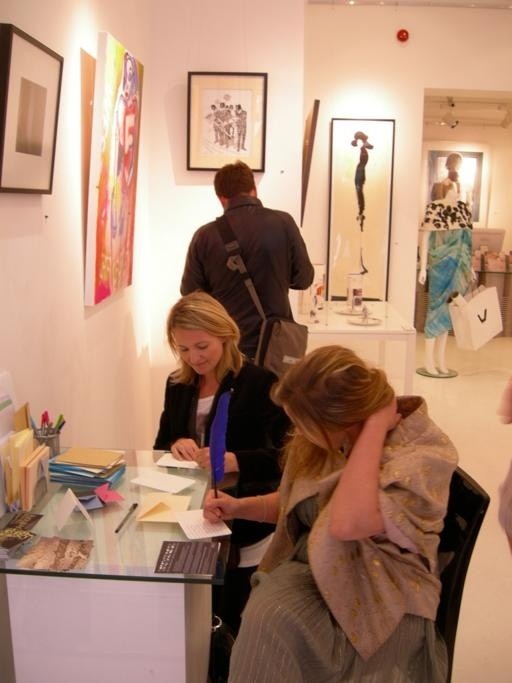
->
xmin=351 ymin=132 xmax=374 ymax=149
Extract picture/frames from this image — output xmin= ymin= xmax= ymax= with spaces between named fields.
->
xmin=0 ymin=447 xmax=229 ymax=683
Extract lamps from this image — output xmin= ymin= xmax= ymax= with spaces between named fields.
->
xmin=436 ymin=468 xmax=489 ymax=681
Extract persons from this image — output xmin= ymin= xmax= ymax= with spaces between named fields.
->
xmin=208 ymin=102 xmax=248 ymax=153
xmin=205 ymin=345 xmax=457 ymax=683
xmin=153 ymin=292 xmax=295 ymax=681
xmin=182 ymin=162 xmax=314 ymax=380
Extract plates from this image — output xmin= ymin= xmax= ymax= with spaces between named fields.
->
xmin=415 ymin=269 xmax=512 ymax=337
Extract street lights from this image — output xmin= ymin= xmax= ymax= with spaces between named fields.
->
xmin=447 ymin=281 xmax=503 ymax=352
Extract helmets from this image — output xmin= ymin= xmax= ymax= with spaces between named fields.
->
xmin=334 ymin=308 xmax=374 ymax=316
xmin=347 ymin=318 xmax=382 ymax=326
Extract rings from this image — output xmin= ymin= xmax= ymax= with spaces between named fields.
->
xmin=29 ymin=411 xmax=66 ymax=446
xmin=115 ymin=503 xmax=138 ymax=533
xmin=211 ymin=465 xmax=223 ymax=522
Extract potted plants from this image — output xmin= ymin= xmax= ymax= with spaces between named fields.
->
xmin=85 ymin=34 xmax=144 ymax=307
xmin=325 ymin=118 xmax=396 ymax=301
xmin=185 ymin=71 xmax=266 ymax=173
xmin=0 ymin=23 xmax=63 ymax=194
xmin=424 ymin=142 xmax=491 ymax=229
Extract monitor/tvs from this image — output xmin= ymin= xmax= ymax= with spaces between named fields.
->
xmin=0 ymin=394 xmax=50 ymax=514
xmin=49 ymin=444 xmax=126 ymax=493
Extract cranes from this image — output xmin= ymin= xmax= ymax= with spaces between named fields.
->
xmin=49 ymin=445 xmax=126 ymax=487
